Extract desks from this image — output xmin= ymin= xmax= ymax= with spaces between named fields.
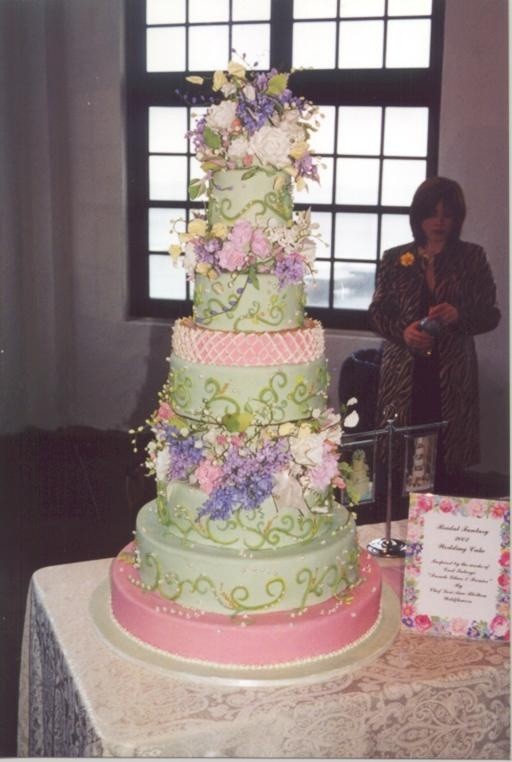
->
xmin=16 ymin=497 xmax=512 ymax=759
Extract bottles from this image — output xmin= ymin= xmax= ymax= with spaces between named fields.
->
xmin=414 ymin=308 xmax=435 ymax=359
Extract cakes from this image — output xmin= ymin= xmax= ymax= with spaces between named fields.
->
xmin=109 ymin=96 xmax=384 ymax=669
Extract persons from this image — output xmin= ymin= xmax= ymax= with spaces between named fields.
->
xmin=367 ymin=174 xmax=502 ymax=523
